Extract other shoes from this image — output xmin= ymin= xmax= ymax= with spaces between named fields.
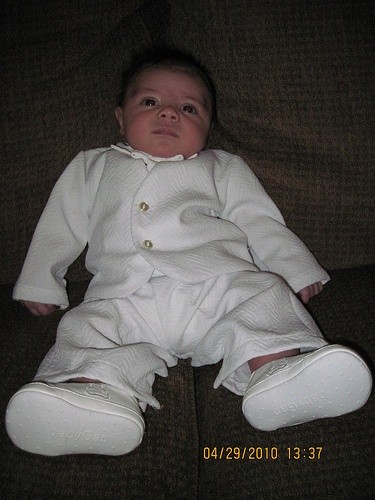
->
xmin=242 ymin=344 xmax=372 ymax=432
xmin=5 ymin=382 xmax=145 ymax=456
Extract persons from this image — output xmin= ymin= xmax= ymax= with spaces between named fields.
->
xmin=6 ymin=49 xmax=373 ymax=456
